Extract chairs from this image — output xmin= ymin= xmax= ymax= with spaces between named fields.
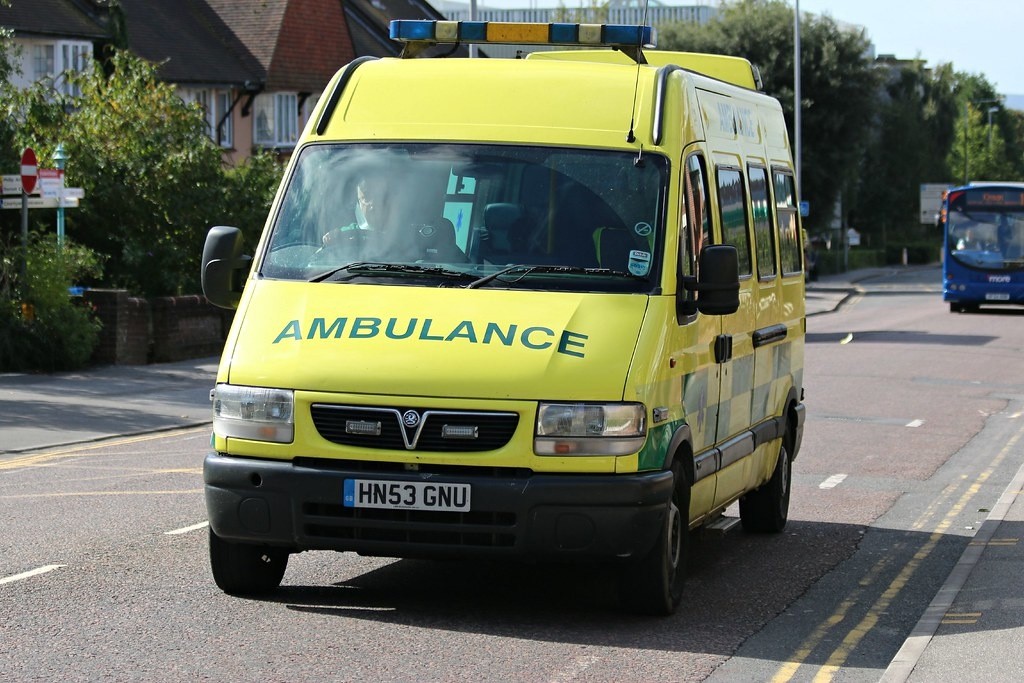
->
xmin=470 ymin=189 xmax=653 ymax=271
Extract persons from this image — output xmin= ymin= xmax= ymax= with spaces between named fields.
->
xmin=322 ymin=171 xmax=470 ymax=263
xmin=957 ymin=227 xmax=983 ymax=251
xmin=997 ymin=214 xmax=1012 ymax=257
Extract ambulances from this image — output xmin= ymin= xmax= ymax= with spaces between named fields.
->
xmin=201 ymin=19 xmax=809 ymax=623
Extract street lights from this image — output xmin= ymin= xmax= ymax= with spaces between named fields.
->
xmin=987 ymin=106 xmax=1000 ymax=142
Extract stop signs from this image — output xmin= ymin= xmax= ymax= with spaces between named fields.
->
xmin=20 ymin=147 xmax=38 ymax=196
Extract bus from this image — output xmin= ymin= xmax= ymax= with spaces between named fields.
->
xmin=935 ymin=180 xmax=1023 ymax=313
